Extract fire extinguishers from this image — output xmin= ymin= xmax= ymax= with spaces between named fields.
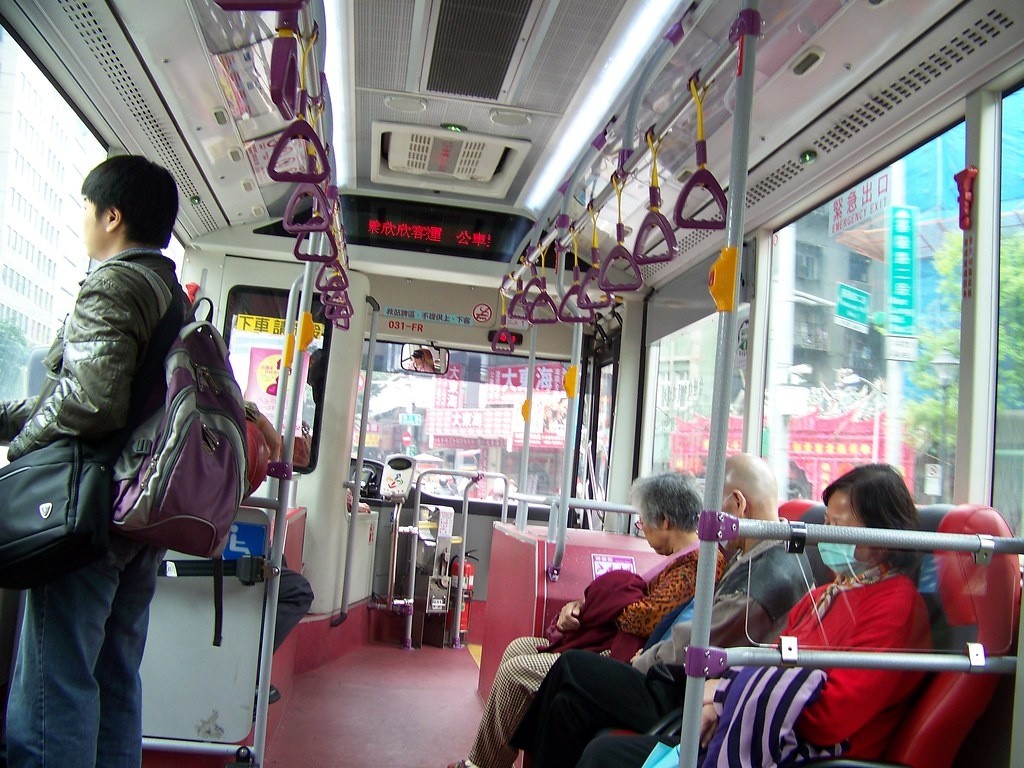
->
xmin=446 ymin=549 xmax=479 ymax=634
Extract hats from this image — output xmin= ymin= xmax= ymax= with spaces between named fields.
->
xmin=411 ymin=350 xmax=423 ymax=358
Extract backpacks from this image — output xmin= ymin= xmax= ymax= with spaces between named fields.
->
xmin=86 ymin=259 xmax=248 ymax=559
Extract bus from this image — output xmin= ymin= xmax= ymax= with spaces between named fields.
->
xmin=24 ymin=345 xmax=51 ymax=398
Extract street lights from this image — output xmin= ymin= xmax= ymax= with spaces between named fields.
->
xmin=844 ymin=374 xmax=881 ymax=463
xmin=931 ymin=352 xmax=960 ymax=507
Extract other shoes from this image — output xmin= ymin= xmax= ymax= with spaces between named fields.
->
xmin=447 ymin=759 xmax=469 ymax=768
xmin=255 ymin=684 xmax=280 ymax=703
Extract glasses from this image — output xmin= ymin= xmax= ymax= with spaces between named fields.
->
xmin=634 ymin=520 xmax=644 ymax=530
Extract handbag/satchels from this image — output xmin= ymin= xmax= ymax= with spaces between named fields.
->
xmin=0 ymin=437 xmax=112 ymax=589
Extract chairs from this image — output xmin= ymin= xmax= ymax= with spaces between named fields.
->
xmin=598 ymin=497 xmax=1021 ymax=768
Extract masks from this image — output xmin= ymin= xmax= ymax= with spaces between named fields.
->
xmin=817 ymin=541 xmax=867 ymax=578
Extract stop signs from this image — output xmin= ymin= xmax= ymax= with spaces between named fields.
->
xmin=402 ymin=432 xmax=412 ymax=447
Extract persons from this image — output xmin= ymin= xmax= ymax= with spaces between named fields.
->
xmin=0 ymin=154 xmax=312 ymax=768
xmin=512 ymin=453 xmax=812 ymax=768
xmin=576 ymin=463 xmax=930 ymax=768
xmin=408 ymin=350 xmax=435 ymax=373
xmin=447 ymin=472 xmax=727 ymax=768
